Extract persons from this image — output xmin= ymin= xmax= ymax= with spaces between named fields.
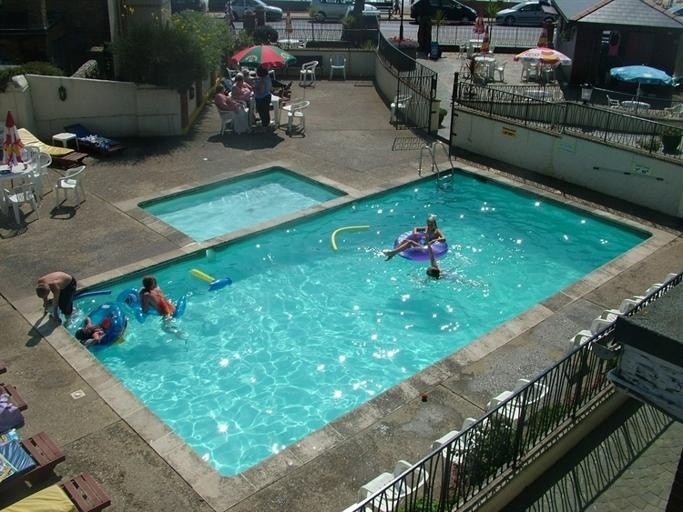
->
xmin=35 ymin=271 xmax=78 ymax=329
xmin=394 ymin=0 xmax=401 ymax=15
xmin=382 ymin=216 xmax=446 ymax=257
xmin=139 ymin=275 xmax=190 ymax=345
xmin=214 ymin=66 xmax=294 ymax=128
xmin=75 ymin=317 xmax=105 ymax=344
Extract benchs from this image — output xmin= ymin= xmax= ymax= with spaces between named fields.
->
xmin=1 ymin=432 xmax=112 ymax=511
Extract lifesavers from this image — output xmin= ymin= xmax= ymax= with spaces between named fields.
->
xmin=82 ymin=302 xmax=124 ymax=345
xmin=394 ymin=231 xmax=447 ymax=260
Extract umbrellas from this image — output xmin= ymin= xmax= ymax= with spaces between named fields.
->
xmin=536 ymin=22 xmax=548 ymax=48
xmin=2 ymin=110 xmax=25 ymax=189
xmin=472 ymin=7 xmax=485 ymax=46
xmin=479 ymin=24 xmax=490 ymax=66
xmin=512 ymin=46 xmax=571 ymax=90
xmin=229 ymin=43 xmax=297 ymax=70
xmin=609 ymin=63 xmax=673 ymax=111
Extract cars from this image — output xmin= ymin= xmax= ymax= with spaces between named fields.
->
xmin=224 ymin=0 xmax=283 ymax=21
xmin=410 ymin=0 xmax=477 ymax=24
xmin=494 ymin=1 xmax=557 ymax=28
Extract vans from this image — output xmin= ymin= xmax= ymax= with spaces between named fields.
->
xmin=308 ymin=0 xmax=377 ymax=23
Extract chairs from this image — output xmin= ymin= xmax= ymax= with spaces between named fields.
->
xmin=2 ymin=123 xmax=127 ymax=224
xmin=457 ymin=42 xmax=509 ymax=82
xmin=389 ymin=94 xmax=412 ymax=124
xmin=214 ymin=91 xmax=310 ymax=138
xmin=606 ymin=94 xmax=619 ymax=109
xmin=298 ymin=60 xmax=319 ymax=89
xmin=329 ymin=54 xmax=347 ymax=81
xmin=520 ymin=60 xmax=553 ymax=83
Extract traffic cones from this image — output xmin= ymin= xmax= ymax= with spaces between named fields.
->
xmin=285 ymin=11 xmax=293 ymax=32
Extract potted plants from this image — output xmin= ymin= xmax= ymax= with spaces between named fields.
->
xmin=660 ymin=126 xmax=682 ymax=153
